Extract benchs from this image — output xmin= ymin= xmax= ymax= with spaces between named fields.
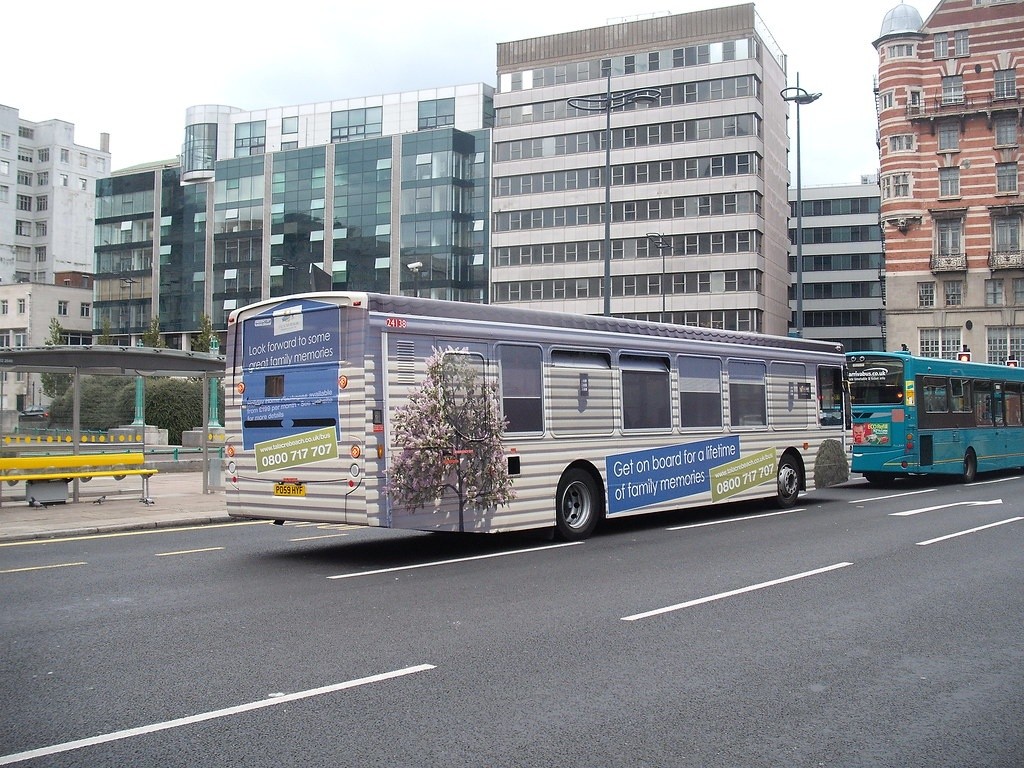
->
xmin=0 ymin=453 xmax=159 ymax=506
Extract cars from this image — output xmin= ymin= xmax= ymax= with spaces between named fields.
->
xmin=18 ymin=405 xmax=49 ymax=422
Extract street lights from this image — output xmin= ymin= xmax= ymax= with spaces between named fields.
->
xmin=566 ymin=74 xmax=662 ymax=318
xmin=780 ymin=71 xmax=823 ymax=340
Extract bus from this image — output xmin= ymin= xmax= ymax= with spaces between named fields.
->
xmin=222 ymin=289 xmax=855 ymax=542
xmin=844 ymin=351 xmax=1024 ymax=484
